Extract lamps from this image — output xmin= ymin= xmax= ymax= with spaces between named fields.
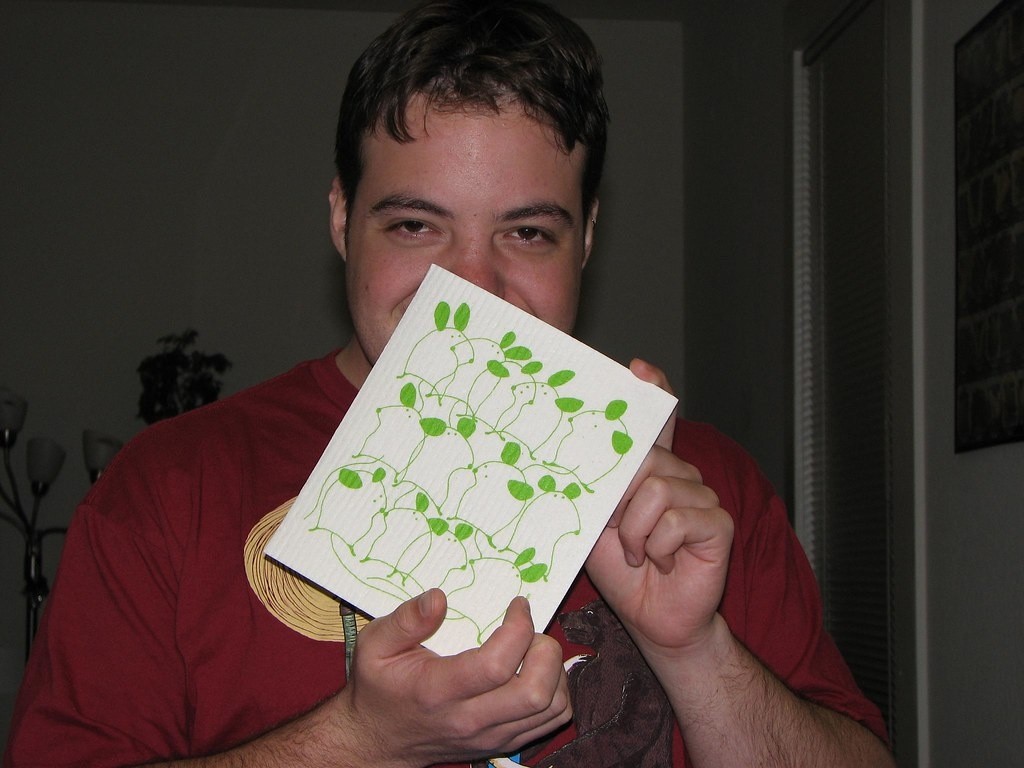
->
xmin=0 ymin=385 xmax=124 ymax=668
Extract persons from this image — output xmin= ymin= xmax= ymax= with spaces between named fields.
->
xmin=0 ymin=0 xmax=895 ymax=768
